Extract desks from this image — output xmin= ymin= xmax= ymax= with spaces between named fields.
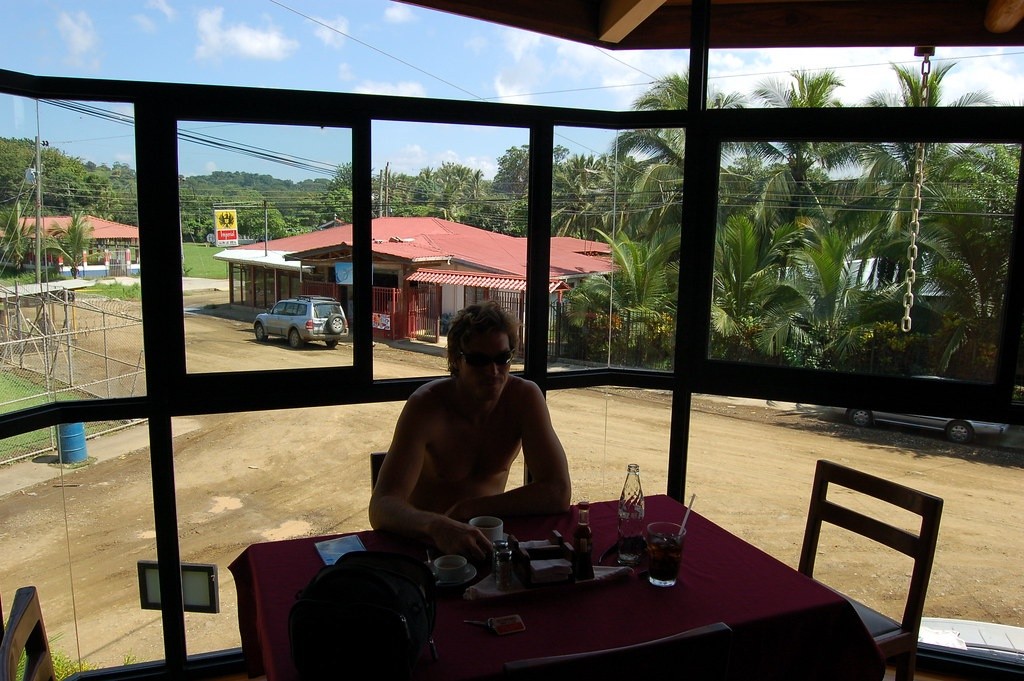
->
xmin=229 ymin=495 xmax=887 ymax=681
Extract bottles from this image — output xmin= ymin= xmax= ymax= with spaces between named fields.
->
xmin=492 ymin=541 xmax=509 ymax=579
xmin=618 ymin=464 xmax=645 ymax=566
xmin=572 ymin=501 xmax=591 ymax=557
xmin=496 ymin=549 xmax=512 ymax=591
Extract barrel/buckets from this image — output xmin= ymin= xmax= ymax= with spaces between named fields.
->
xmin=55 ymin=421 xmax=86 ymax=464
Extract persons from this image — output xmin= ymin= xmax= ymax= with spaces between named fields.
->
xmin=370 ymin=301 xmax=571 ymax=561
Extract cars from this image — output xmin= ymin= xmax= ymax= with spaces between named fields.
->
xmin=845 ymin=375 xmax=1011 ymax=444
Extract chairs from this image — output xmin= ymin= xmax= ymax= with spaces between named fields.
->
xmin=796 ymin=460 xmax=943 ymax=681
xmin=0 ymin=587 xmax=64 ymax=681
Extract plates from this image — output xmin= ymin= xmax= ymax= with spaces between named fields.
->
xmin=424 ymin=560 xmax=477 ymax=585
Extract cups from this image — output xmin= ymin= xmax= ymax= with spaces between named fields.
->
xmin=468 ymin=516 xmax=503 ymax=551
xmin=647 ymin=522 xmax=686 ymax=586
xmin=433 ymin=555 xmax=467 ymax=581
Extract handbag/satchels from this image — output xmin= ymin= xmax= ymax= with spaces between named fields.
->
xmin=286 ymin=549 xmax=438 ymax=681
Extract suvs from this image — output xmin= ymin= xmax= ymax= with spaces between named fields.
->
xmin=252 ymin=294 xmax=349 ymax=350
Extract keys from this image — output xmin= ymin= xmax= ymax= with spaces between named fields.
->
xmin=464 ymin=614 xmax=526 ymax=635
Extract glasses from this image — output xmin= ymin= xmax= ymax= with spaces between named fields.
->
xmin=456 ymin=346 xmax=515 ymax=366
